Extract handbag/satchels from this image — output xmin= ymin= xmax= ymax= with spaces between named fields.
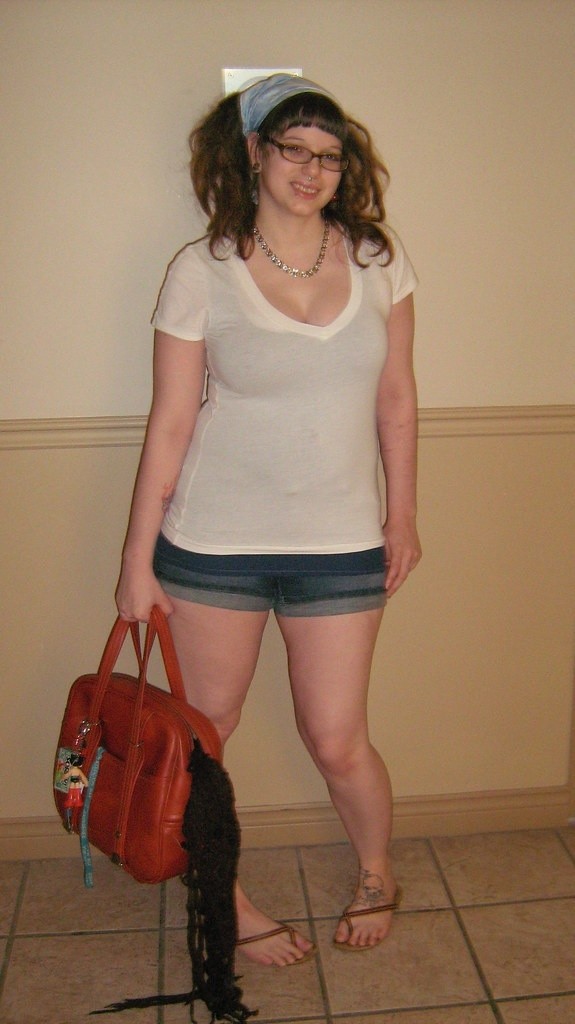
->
xmin=52 ymin=604 xmax=224 ymax=885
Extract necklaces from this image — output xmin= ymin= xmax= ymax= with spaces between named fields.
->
xmin=250 ymin=216 xmax=331 ymax=279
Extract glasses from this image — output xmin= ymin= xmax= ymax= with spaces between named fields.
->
xmin=260 ymin=131 xmax=349 ymax=171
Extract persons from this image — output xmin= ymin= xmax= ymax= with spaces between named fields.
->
xmin=114 ymin=73 xmax=422 ymax=967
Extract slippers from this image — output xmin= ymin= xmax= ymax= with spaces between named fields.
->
xmin=233 ymin=920 xmax=320 ymax=965
xmin=333 ymin=884 xmax=402 ymax=951
